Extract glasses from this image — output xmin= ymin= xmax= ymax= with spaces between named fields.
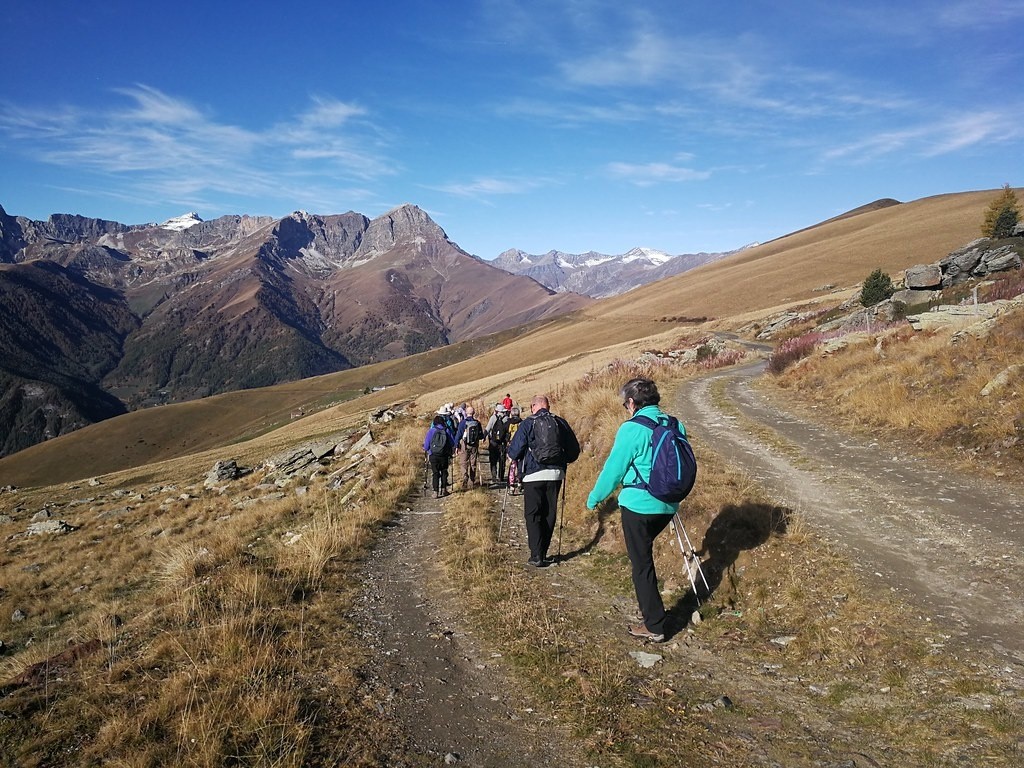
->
xmin=623 ymin=401 xmax=629 ymax=409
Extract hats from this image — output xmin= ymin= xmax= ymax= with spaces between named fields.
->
xmin=494 ymin=405 xmax=508 ymax=412
xmin=436 ymin=403 xmax=474 ymax=415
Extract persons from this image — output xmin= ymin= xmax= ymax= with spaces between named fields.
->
xmin=508 ymin=396 xmax=580 ymax=567
xmin=586 ymin=379 xmax=686 ymax=642
xmin=424 ymin=394 xmax=524 ymax=496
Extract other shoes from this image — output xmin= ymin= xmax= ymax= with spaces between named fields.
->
xmin=517 ymin=486 xmax=522 ymax=492
xmin=508 ymin=489 xmax=515 ymax=494
xmin=497 ymin=477 xmax=504 ymax=482
xmin=442 ymin=489 xmax=449 ymax=496
xmin=470 ymin=478 xmax=475 ymax=483
xmin=432 ymin=492 xmax=437 ymax=499
xmin=492 ymin=471 xmax=497 ymax=482
xmin=462 ymin=474 xmax=468 ymax=487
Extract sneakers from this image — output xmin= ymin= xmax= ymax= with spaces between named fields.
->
xmin=527 ymin=555 xmax=546 ymax=567
xmin=629 ymin=623 xmax=664 ymax=643
xmin=630 ymin=611 xmax=646 ymax=624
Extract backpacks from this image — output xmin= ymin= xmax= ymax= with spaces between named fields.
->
xmin=492 ymin=415 xmax=507 ymax=441
xmin=462 ymin=419 xmax=480 ymax=447
xmin=508 ymin=420 xmax=520 ymax=441
xmin=624 ymin=415 xmax=698 ymax=504
xmin=443 ymin=416 xmax=455 ymax=434
xmin=528 ymin=414 xmax=567 ymax=465
xmin=431 ymin=427 xmax=451 ymax=456
